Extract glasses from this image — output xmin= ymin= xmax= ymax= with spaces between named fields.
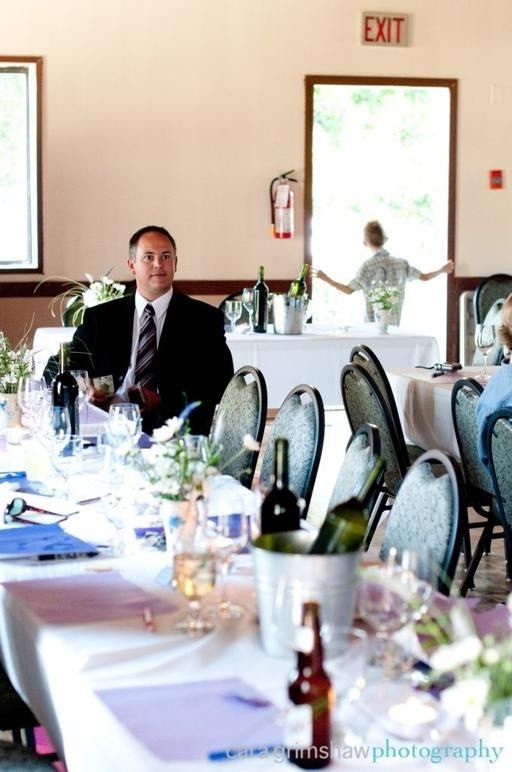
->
xmin=3 ymin=496 xmax=81 ymax=528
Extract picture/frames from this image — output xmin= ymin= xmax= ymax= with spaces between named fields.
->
xmin=0 ymin=54 xmax=44 ymax=274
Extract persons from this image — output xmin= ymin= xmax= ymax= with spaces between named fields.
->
xmin=309 ymin=221 xmax=455 ymax=327
xmin=474 ymin=295 xmax=512 ymax=508
xmin=45 ymin=225 xmax=235 ymax=436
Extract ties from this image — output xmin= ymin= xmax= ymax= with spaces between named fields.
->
xmin=136 ymin=304 xmax=157 ymax=391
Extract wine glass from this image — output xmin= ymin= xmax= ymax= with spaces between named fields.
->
xmin=473 ymin=322 xmax=498 ymax=384
xmin=224 ymin=287 xmax=256 ymax=334
xmin=16 ymin=371 xmax=249 ymax=638
xmin=359 ymin=547 xmax=443 ymax=683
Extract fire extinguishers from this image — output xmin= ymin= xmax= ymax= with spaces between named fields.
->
xmin=270 ymin=169 xmax=298 ymax=239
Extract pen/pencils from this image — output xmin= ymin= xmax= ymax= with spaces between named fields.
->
xmin=209 ymin=746 xmax=280 ymax=760
xmin=143 ymin=608 xmax=155 ymax=633
xmin=78 ymin=496 xmax=101 ymax=505
xmin=30 ymin=552 xmax=99 ymax=562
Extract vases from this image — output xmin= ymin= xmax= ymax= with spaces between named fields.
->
xmin=148 ymin=501 xmax=208 ymax=555
xmin=373 ymin=311 xmax=392 ymax=334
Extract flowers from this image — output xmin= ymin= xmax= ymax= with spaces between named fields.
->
xmin=123 ymin=416 xmax=263 ymax=501
xmin=32 ymin=262 xmax=127 ymax=329
xmin=367 ymin=280 xmax=402 ymax=310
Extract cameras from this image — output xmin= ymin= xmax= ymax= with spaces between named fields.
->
xmin=434 ymin=362 xmax=462 ymax=374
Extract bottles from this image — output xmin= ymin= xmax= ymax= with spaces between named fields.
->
xmin=288 ymin=263 xmax=310 ymax=300
xmin=260 ymin=439 xmax=301 ymax=533
xmin=305 ymin=455 xmax=388 ymax=562
xmin=253 ymin=264 xmax=268 ymax=334
xmin=279 ymin=599 xmax=337 ymax=769
xmin=50 ymin=340 xmax=80 ymax=441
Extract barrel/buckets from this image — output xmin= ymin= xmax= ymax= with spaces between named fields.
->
xmin=271 ymin=293 xmax=307 ymax=334
xmin=247 ymin=539 xmax=363 ymax=669
